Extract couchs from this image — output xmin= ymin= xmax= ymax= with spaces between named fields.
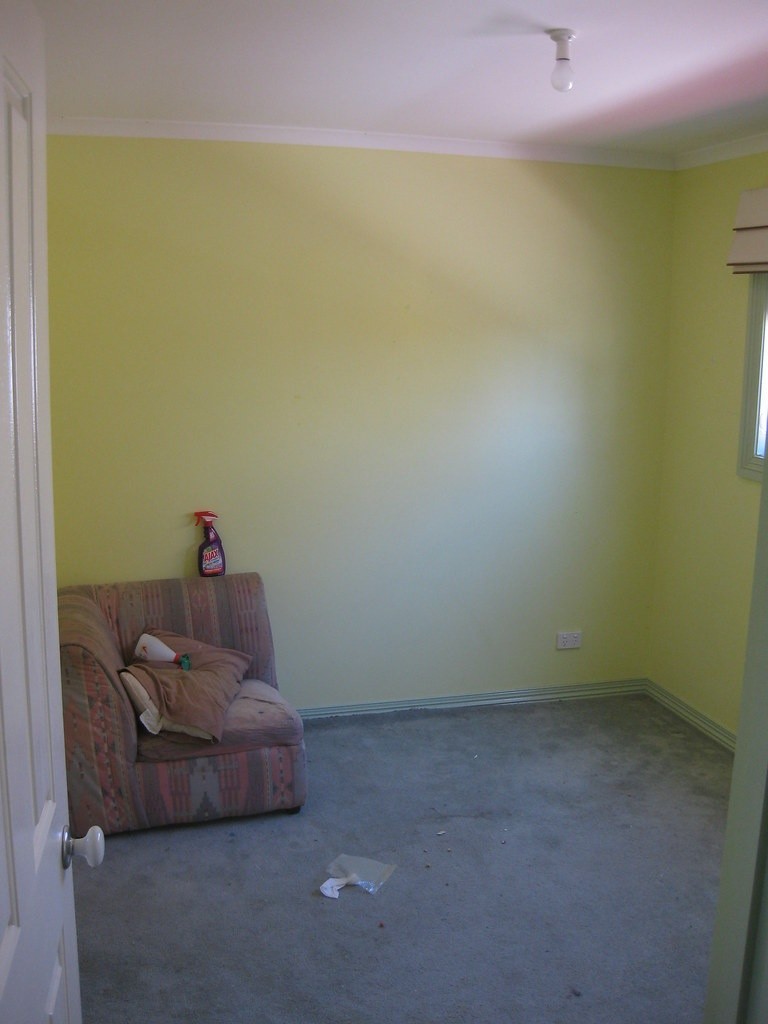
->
xmin=55 ymin=570 xmax=308 ymax=838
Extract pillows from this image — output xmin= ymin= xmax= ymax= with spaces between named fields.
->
xmin=118 ymin=626 xmax=254 ymax=744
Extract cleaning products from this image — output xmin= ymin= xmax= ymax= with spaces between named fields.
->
xmin=192 ymin=511 xmax=225 ymax=577
xmin=135 ymin=633 xmax=192 ymax=672
